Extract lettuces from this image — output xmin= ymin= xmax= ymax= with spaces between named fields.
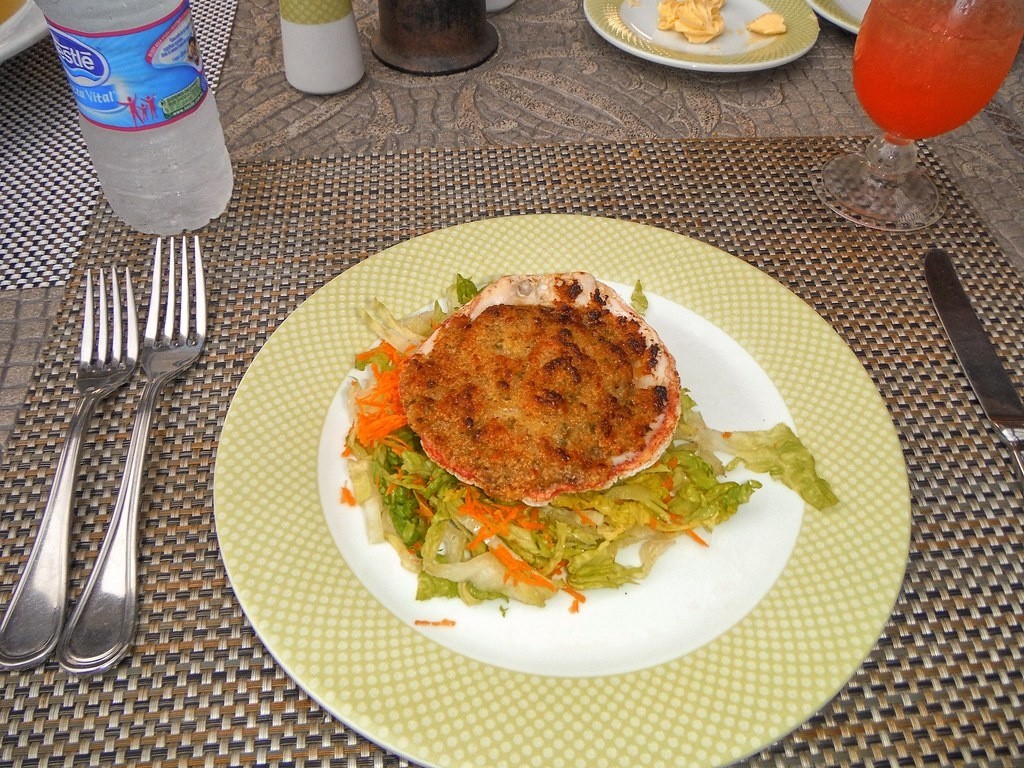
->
xmin=342 ymin=272 xmax=839 ymax=617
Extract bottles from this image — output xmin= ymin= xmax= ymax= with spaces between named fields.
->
xmin=34 ymin=0 xmax=234 ymax=235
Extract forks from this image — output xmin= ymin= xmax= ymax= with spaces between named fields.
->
xmin=0 ymin=268 xmax=139 ymax=671
xmin=54 ymin=235 xmax=207 ymax=678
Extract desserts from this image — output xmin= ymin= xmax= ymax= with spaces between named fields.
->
xmin=659 ymin=0 xmax=727 ymax=43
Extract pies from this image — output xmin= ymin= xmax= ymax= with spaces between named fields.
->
xmin=399 ymin=272 xmax=683 ymax=508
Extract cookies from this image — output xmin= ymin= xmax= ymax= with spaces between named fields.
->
xmin=747 ymin=13 xmax=787 ymax=35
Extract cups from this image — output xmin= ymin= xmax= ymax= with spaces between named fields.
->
xmin=277 ymin=0 xmax=364 ymax=95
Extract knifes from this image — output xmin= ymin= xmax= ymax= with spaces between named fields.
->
xmin=924 ymin=247 xmax=1024 ymax=481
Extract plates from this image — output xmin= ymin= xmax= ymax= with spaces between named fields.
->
xmin=808 ymin=0 xmax=871 ymax=33
xmin=211 ymin=213 xmax=911 ymax=768
xmin=0 ymin=0 xmax=48 ymax=62
xmin=583 ymin=0 xmax=819 ymax=72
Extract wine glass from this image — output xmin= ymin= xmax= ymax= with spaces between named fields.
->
xmin=810 ymin=0 xmax=1024 ymax=231
